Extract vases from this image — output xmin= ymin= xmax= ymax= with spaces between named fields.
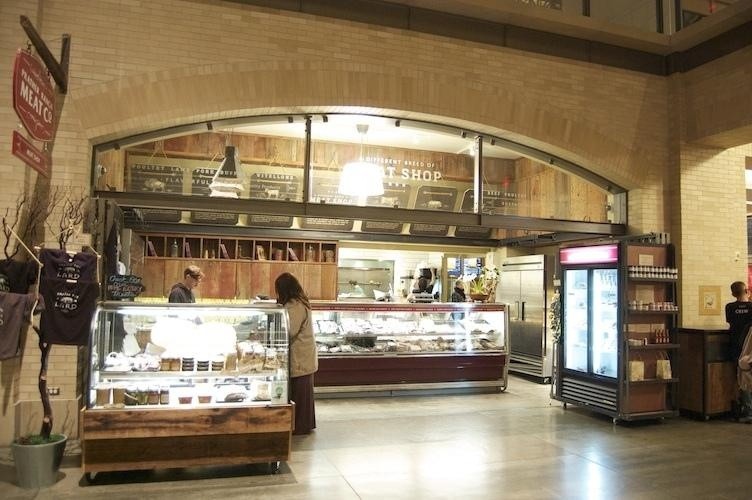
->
xmin=469 ymin=294 xmax=488 ymax=302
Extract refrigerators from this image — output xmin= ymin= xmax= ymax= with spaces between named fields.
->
xmin=555 ymin=239 xmax=671 ymax=425
xmin=497 ymin=253 xmax=555 ymax=383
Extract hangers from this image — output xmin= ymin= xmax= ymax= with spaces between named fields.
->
xmin=33 ymin=218 xmax=102 ymax=259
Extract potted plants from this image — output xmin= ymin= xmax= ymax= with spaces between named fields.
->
xmin=0 ymin=186 xmax=103 ymax=491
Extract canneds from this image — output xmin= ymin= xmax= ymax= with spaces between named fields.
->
xmin=160 ymin=357 xmax=181 ymax=371
xmin=125 ymin=386 xmax=170 ymax=406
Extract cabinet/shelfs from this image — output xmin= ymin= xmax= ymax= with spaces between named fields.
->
xmin=79 ymin=299 xmax=296 ymax=482
xmin=312 ymin=302 xmax=508 ymax=398
xmin=678 ymin=328 xmax=737 ymax=421
xmin=627 ymin=275 xmax=680 ymax=389
xmin=130 ymin=232 xmax=339 ymax=301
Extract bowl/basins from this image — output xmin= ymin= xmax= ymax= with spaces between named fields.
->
xmin=198 ymin=396 xmax=212 ymax=403
xmin=178 ymin=397 xmax=193 ymax=404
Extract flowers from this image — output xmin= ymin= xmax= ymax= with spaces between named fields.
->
xmin=463 ymin=264 xmax=500 ymax=294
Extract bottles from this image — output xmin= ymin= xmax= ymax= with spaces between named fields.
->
xmin=306 ymin=244 xmax=315 ymax=262
xmin=171 ymin=238 xmax=178 ymax=256
xmin=625 ymin=263 xmax=679 ymax=346
xmin=203 ymin=240 xmax=209 ymax=259
xmin=212 ymin=242 xmax=216 ymax=258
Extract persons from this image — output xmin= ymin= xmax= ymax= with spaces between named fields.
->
xmin=452 ymin=281 xmax=466 ymax=303
xmin=275 ymin=272 xmax=318 ymax=434
xmin=169 ymin=266 xmax=204 ymax=302
xmin=726 ymin=281 xmax=752 ymax=421
xmin=413 ymin=267 xmax=436 ymax=303
xmin=349 ymin=279 xmax=364 ymax=294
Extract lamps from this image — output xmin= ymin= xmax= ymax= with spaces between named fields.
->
xmin=209 ymin=129 xmax=249 ymax=195
xmin=337 ymin=121 xmax=384 ymax=198
